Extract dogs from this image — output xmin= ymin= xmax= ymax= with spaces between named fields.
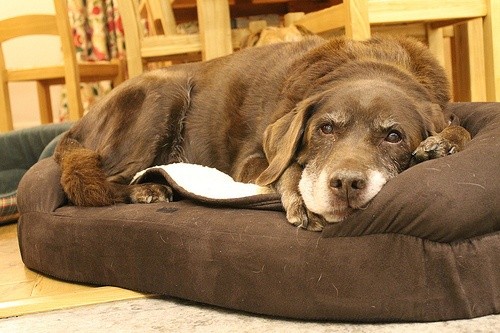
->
xmin=52 ymin=32 xmax=472 ymax=231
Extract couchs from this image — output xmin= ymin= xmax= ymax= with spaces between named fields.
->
xmin=16 ymin=92 xmax=500 ymax=323
